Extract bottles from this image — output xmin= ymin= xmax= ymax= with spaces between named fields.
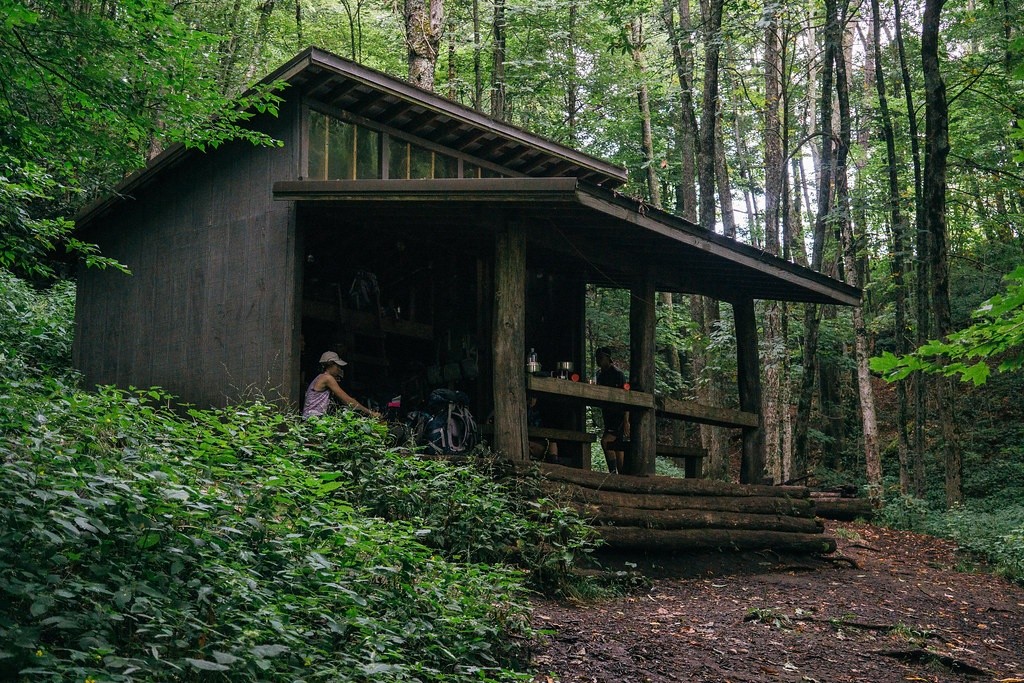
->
xmin=526 ymin=348 xmax=538 ymax=362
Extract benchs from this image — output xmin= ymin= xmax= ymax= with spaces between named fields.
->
xmin=606 ymin=440 xmax=709 ymax=479
xmin=485 ymin=424 xmax=597 ymax=470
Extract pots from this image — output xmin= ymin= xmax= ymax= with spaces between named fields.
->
xmin=554 ymin=361 xmax=574 ymax=372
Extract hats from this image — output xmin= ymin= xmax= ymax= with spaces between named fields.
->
xmin=319 ymin=351 xmax=348 ymax=367
xmin=596 ymin=347 xmax=613 ymax=363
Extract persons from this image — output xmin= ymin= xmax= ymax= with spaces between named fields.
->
xmin=301 ymin=350 xmax=384 ymax=421
xmin=332 ymin=336 xmax=353 ymax=391
xmin=300 ymin=332 xmax=305 ymax=385
xmin=596 ymin=347 xmax=630 ymax=474
xmin=526 ymin=390 xmax=558 ymax=464
